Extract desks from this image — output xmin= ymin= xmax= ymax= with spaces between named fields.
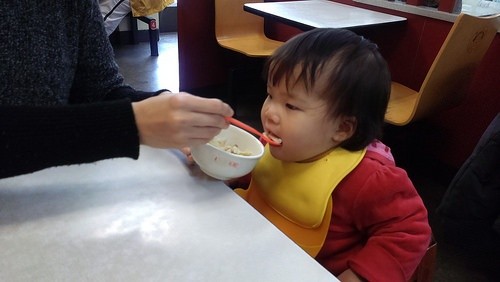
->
xmin=0 ymin=143 xmax=343 ymax=282
xmin=242 ymin=0 xmax=407 ymax=35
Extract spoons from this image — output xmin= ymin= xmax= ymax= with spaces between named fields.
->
xmin=224 ymin=116 xmax=281 ymax=148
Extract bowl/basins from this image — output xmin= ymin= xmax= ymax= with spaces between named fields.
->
xmin=191 ymin=124 xmax=265 ymax=180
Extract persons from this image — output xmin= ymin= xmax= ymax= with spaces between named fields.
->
xmin=0 ymin=0 xmax=234 ymax=180
xmin=181 ymin=27 xmax=432 ymax=282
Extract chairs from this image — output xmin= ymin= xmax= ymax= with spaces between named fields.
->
xmin=215 ymin=0 xmax=286 ymax=57
xmin=382 ymin=11 xmax=500 ymax=125
